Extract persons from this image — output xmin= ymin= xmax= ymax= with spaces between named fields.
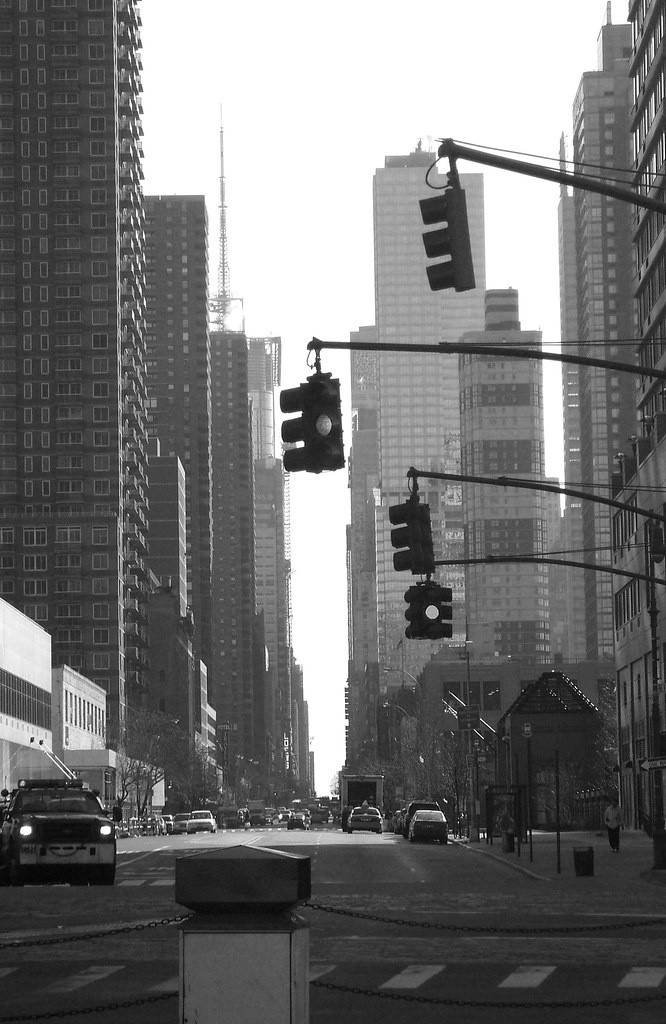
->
xmin=243 ymin=810 xmax=249 ymax=830
xmin=385 ymin=809 xmax=393 ymax=832
xmin=605 ymin=799 xmax=624 ymax=852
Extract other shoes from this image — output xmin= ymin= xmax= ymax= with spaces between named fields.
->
xmin=614 ymin=848 xmax=618 ymax=853
xmin=611 ymin=847 xmax=614 ymax=851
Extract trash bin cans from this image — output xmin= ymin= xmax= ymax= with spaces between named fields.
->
xmin=572 ymin=846 xmax=597 ymax=881
xmin=502 ymin=832 xmax=515 ymax=854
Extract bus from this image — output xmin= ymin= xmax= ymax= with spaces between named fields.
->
xmin=317 ymin=805 xmax=329 ymax=822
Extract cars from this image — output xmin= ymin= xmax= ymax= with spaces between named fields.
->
xmin=391 ymin=809 xmax=405 ymax=833
xmin=187 ymin=810 xmax=217 ymax=834
xmin=311 ymin=814 xmax=322 ymax=824
xmin=347 ymin=806 xmax=383 ymax=833
xmin=406 ymin=810 xmax=447 ymax=843
xmin=265 ymin=806 xmax=312 ymax=830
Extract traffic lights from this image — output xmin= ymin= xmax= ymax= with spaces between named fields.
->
xmin=280 ymin=373 xmax=347 ymax=473
xmin=403 ymin=585 xmax=453 ymax=640
xmin=389 ymin=501 xmax=435 ymax=575
xmin=419 ymin=188 xmax=476 ymax=293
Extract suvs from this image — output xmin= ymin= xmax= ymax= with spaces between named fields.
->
xmin=0 ymin=779 xmax=125 ymax=886
xmin=173 ymin=812 xmax=192 ymax=834
xmin=403 ymin=801 xmax=441 ymax=838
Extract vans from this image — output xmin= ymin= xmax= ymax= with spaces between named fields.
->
xmin=160 ymin=815 xmax=173 ymax=833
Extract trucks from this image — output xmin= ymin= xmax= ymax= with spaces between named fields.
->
xmin=246 ymin=800 xmax=265 ymax=825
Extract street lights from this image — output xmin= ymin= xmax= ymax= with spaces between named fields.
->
xmin=384 ymin=667 xmax=433 ymax=801
